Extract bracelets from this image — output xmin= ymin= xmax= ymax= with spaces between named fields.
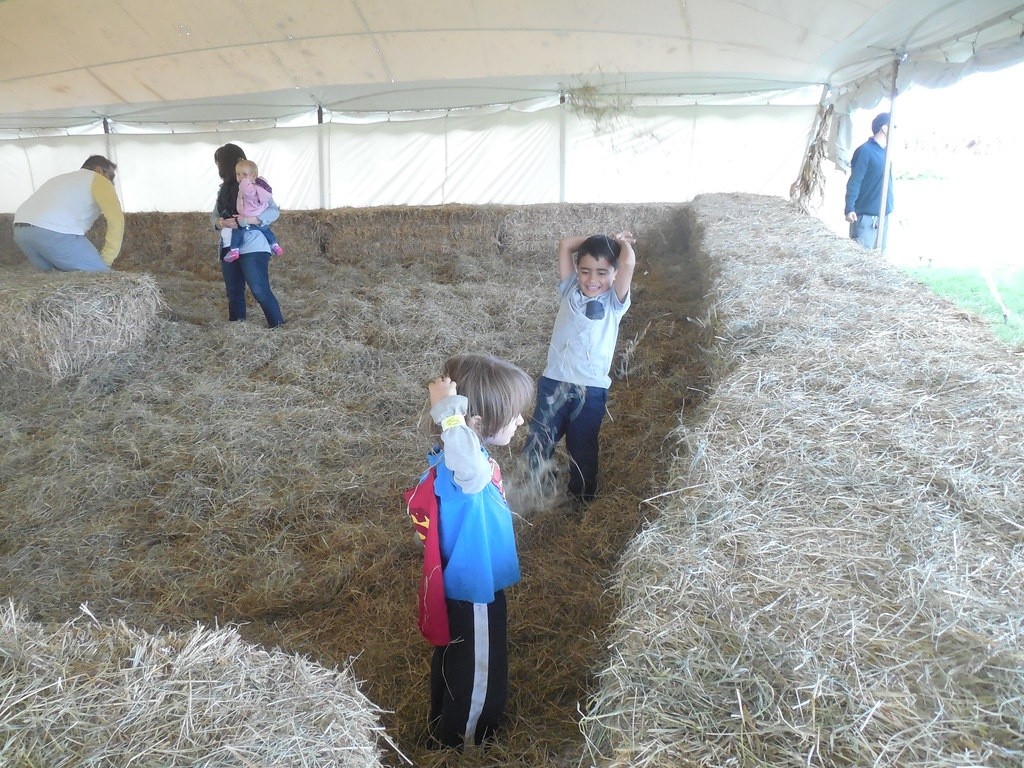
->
xmin=441 ymin=415 xmax=466 ymax=431
xmin=245 ymin=218 xmax=250 ymax=230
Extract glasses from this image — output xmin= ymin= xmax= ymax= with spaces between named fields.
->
xmin=103 ymin=168 xmax=115 ymax=180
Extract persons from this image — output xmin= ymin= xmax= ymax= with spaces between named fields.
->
xmin=13 ymin=154 xmax=125 ymax=272
xmin=224 ymin=160 xmax=283 ymax=262
xmin=402 ymin=354 xmax=535 ymax=750
xmin=524 ymin=232 xmax=636 ymax=500
xmin=845 ymin=113 xmax=894 ymax=253
xmin=210 ymin=143 xmax=284 ymax=328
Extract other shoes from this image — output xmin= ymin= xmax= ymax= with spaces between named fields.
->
xmin=224 ymin=251 xmax=240 ymax=263
xmin=273 ymin=245 xmax=283 ymax=256
